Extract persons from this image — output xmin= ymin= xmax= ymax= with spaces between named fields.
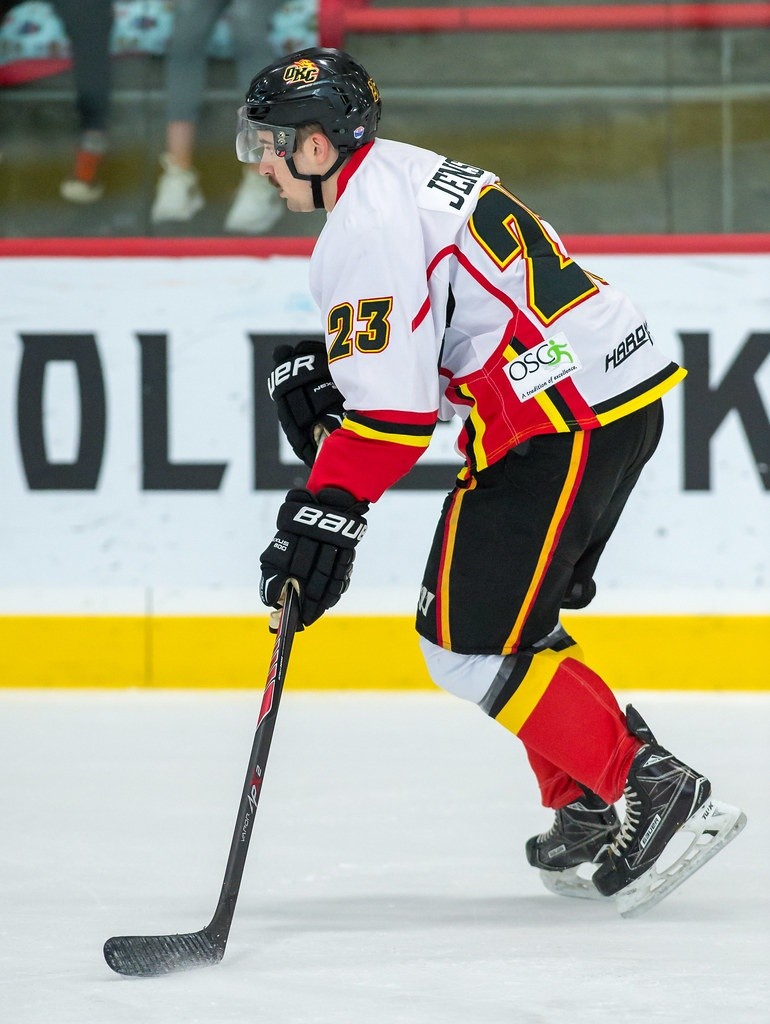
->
xmin=151 ymin=0 xmax=285 ymax=237
xmin=235 ymin=48 xmax=751 ymax=918
xmin=0 ymin=0 xmax=115 ymax=203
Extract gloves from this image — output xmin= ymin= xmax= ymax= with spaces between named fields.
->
xmin=269 ymin=332 xmax=348 ymax=463
xmin=256 ymin=484 xmax=368 ymax=630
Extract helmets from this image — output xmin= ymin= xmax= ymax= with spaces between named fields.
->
xmin=245 ymin=46 xmax=383 ymax=148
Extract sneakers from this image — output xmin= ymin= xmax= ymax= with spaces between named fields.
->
xmin=225 ymin=169 xmax=289 ymax=234
xmin=523 ymin=786 xmax=621 ymax=904
xmin=58 ymin=146 xmax=110 ymax=201
xmin=594 ymin=706 xmax=749 ymax=919
xmin=150 ymin=154 xmax=205 ymax=223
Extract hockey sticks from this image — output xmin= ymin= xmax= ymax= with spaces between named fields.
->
xmin=101 ymin=426 xmax=327 ymax=979
